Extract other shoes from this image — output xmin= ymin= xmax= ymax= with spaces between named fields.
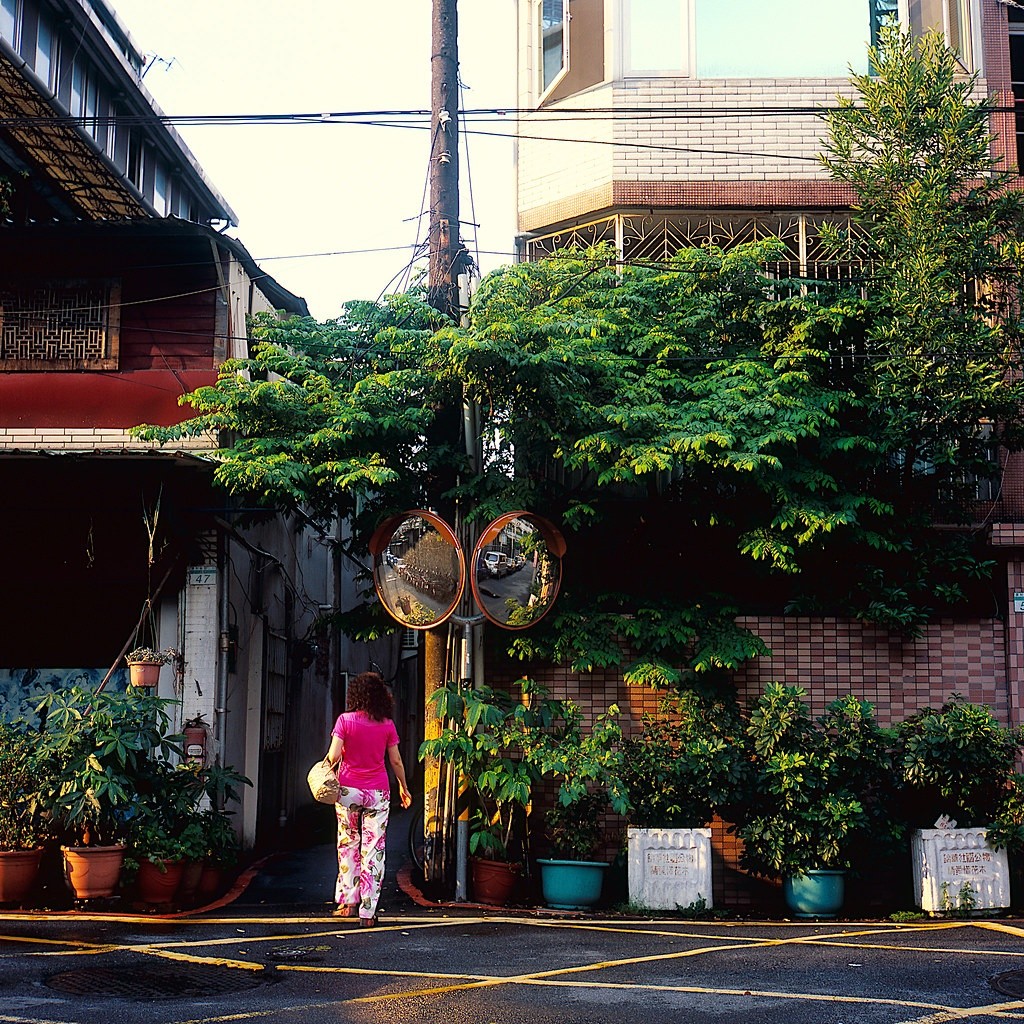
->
xmin=359 ymin=915 xmax=378 ymax=929
xmin=332 ymin=905 xmax=355 ymax=917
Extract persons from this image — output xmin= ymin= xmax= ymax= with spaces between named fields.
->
xmin=328 ymin=672 xmax=411 ymax=926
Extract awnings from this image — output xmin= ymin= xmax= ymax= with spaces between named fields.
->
xmin=1 ymin=447 xmax=221 ymax=467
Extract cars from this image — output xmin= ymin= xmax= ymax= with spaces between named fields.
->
xmin=485 ymin=551 xmax=526 ymax=579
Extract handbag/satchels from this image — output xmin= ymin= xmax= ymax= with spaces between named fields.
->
xmin=306 ymin=753 xmax=342 ymax=804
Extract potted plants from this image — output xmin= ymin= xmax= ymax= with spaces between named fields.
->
xmin=395 ymin=596 xmax=412 ymax=615
xmin=0 ymin=645 xmax=254 ymax=902
xmin=417 ymin=678 xmax=1024 ymax=920
